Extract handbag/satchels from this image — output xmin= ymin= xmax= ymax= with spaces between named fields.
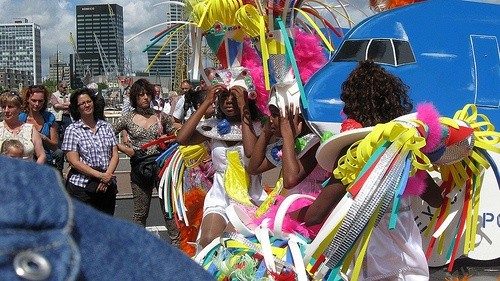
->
xmin=130 ymin=149 xmax=165 ymax=184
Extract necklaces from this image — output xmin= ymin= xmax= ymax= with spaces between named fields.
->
xmin=140 ymin=114 xmax=150 ymax=125
xmin=304 ymin=61 xmax=432 ymax=281
xmin=88 ymin=122 xmax=95 ymax=128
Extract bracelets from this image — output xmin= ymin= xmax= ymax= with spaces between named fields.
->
xmin=101 ymin=172 xmax=104 ymax=182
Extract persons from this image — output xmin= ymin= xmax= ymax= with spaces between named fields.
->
xmin=19 ymin=85 xmax=58 ymax=165
xmin=153 ymin=68 xmax=323 ymax=262
xmin=123 ymin=88 xmax=135 ymax=146
xmin=50 ymin=79 xmax=72 ymax=162
xmin=1 ymin=140 xmax=24 ymax=158
xmin=61 ymin=91 xmax=119 ymax=216
xmin=113 ymin=78 xmax=180 ymax=247
xmin=0 ymin=92 xmax=47 ymax=166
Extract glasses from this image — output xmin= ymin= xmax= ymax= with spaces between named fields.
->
xmin=30 ymin=84 xmax=45 ymax=89
xmin=62 ymin=87 xmax=68 ymax=89
xmin=1 ymin=90 xmax=21 ymax=99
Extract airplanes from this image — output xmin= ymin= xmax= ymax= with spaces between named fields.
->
xmin=298 ymin=0 xmax=500 ymax=268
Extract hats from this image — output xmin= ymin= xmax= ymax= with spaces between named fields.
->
xmin=87 ymin=82 xmax=99 ymax=95
